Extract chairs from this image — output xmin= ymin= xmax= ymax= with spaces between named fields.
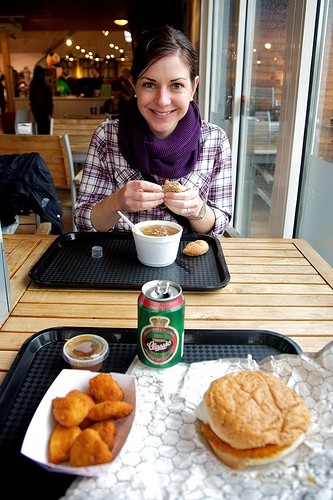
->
xmin=0 ymin=118 xmax=108 ymax=235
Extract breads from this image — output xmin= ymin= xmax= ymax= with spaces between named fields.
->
xmin=182 ymin=240 xmax=209 ymax=256
xmin=161 ymin=181 xmax=181 ymax=193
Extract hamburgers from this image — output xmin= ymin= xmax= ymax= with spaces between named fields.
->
xmin=196 ymin=371 xmax=312 ymax=468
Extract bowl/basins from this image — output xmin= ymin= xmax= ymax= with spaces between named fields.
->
xmin=132 ymin=220 xmax=184 ymax=267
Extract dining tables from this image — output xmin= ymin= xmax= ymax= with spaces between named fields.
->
xmin=0 ymin=235 xmax=333 ymax=500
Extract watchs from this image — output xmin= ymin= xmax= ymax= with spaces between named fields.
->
xmin=189 ymin=203 xmax=207 ymax=220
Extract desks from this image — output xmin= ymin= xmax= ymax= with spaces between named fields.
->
xmin=14 ymin=97 xmax=114 ymax=127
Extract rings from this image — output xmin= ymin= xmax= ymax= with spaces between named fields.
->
xmin=187 ymin=209 xmax=189 ymax=214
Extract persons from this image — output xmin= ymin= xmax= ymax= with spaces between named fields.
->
xmin=101 ymin=79 xmax=128 ymax=115
xmin=0 ymin=69 xmax=7 ymax=114
xmin=29 ymin=64 xmax=54 ymax=136
xmin=116 ymin=68 xmax=131 ymax=97
xmin=56 ymin=76 xmax=71 ymax=96
xmin=71 ymin=24 xmax=234 ymax=239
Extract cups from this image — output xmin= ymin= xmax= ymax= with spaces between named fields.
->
xmin=63 ymin=334 xmax=107 ymax=372
xmin=90 ymin=107 xmax=97 ymax=115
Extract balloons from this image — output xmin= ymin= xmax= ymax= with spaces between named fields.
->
xmin=52 ymin=54 xmax=60 ymax=63
xmin=46 ymin=56 xmax=53 ymax=65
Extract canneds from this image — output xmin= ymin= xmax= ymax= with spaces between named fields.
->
xmin=137 ymin=279 xmax=185 ymax=368
